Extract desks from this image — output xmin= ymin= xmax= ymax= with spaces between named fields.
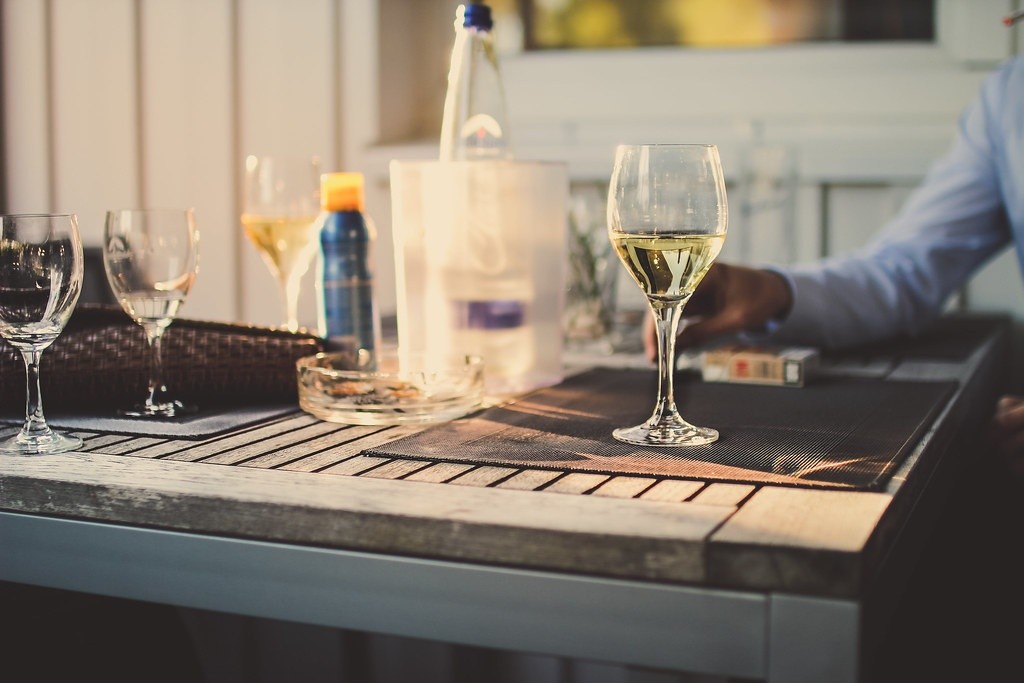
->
xmin=0 ymin=305 xmax=1022 ymax=683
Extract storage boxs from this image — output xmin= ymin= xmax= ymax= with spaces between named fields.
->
xmin=701 ymin=345 xmax=821 ymax=387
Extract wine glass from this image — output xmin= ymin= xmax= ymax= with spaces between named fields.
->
xmin=607 ymin=145 xmax=729 ymax=446
xmin=238 ymin=155 xmax=320 ymax=332
xmin=104 ymin=207 xmax=205 ymax=416
xmin=0 ymin=212 xmax=83 ymax=456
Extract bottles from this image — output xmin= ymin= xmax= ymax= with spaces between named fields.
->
xmin=429 ymin=1 xmax=528 ymax=383
xmin=316 ymin=171 xmax=380 ymax=369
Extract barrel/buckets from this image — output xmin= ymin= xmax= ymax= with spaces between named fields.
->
xmin=391 ymin=159 xmax=566 ymax=385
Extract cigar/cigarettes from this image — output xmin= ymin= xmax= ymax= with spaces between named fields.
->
xmin=1003 ymin=9 xmax=1023 ymax=26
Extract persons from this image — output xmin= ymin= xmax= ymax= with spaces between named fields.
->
xmin=641 ymin=52 xmax=1023 ymax=468
